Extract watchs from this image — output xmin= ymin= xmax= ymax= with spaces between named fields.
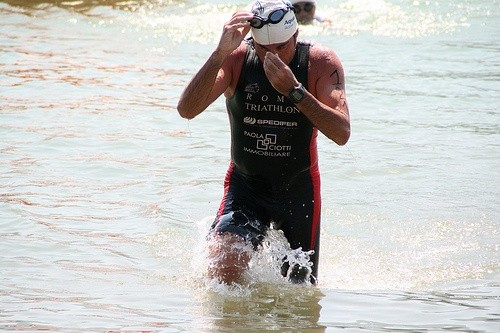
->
xmin=288 ymin=81 xmax=305 ymax=103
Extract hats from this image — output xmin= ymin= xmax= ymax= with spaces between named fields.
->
xmin=289 ymin=0 xmax=315 ymax=4
xmin=250 ymin=0 xmax=300 ymax=45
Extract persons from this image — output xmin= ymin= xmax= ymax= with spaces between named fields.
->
xmin=176 ymin=0 xmax=350 ymax=287
xmin=286 ymin=0 xmax=332 ymax=28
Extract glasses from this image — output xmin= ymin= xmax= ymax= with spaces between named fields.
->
xmin=248 ymin=5 xmax=294 ymax=29
xmin=293 ymin=2 xmax=313 ymax=13
